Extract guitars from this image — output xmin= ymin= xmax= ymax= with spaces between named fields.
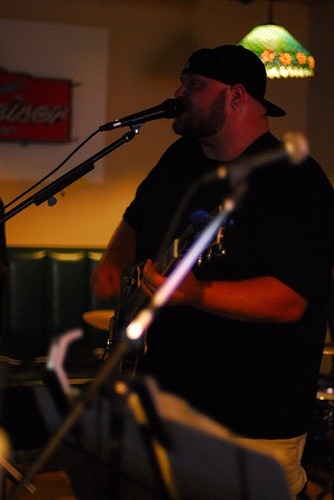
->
xmin=105 ymin=205 xmax=226 ymax=381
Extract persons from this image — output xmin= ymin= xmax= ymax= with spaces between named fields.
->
xmin=91 ymin=45 xmax=333 ymax=500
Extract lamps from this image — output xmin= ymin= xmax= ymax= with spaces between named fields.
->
xmin=237 ymin=0 xmax=316 ymax=79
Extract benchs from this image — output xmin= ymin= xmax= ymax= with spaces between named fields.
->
xmin=0 ymin=247 xmax=154 ymax=451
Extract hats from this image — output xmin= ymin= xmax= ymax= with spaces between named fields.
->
xmin=182 ymin=44 xmax=286 ymax=117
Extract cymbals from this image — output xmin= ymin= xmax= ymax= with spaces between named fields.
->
xmin=82 ymin=309 xmax=115 ymax=331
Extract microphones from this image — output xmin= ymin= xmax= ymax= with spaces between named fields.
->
xmin=100 ymin=98 xmax=184 ymax=131
